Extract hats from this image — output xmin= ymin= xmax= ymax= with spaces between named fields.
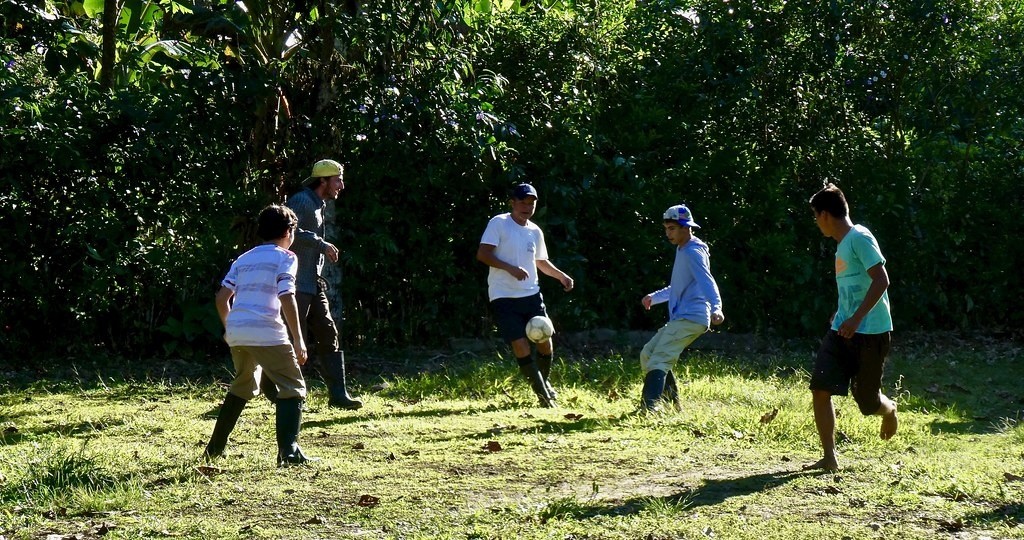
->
xmin=301 ymin=158 xmax=344 ymax=183
xmin=513 ymin=183 xmax=539 ymax=200
xmin=662 ymin=204 xmax=701 ymax=230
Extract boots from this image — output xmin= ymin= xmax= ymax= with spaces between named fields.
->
xmin=628 ymin=369 xmax=680 ymax=417
xmin=520 ymin=357 xmax=556 ymax=408
xmin=260 ymin=370 xmax=279 ymax=404
xmin=202 ymin=391 xmax=248 ymax=463
xmin=320 ymin=350 xmax=362 ymax=411
xmin=275 ymin=396 xmax=321 ymax=468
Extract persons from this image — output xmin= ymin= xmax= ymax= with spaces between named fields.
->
xmin=802 ymin=183 xmax=897 ymax=470
xmin=476 ymin=184 xmax=574 ymax=408
xmin=629 ymin=205 xmax=725 ymax=416
xmin=261 ymin=159 xmax=362 ymax=410
xmin=202 ymin=202 xmax=313 ymax=468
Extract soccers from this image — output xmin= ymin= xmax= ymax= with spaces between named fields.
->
xmin=526 ymin=315 xmax=554 ymax=345
